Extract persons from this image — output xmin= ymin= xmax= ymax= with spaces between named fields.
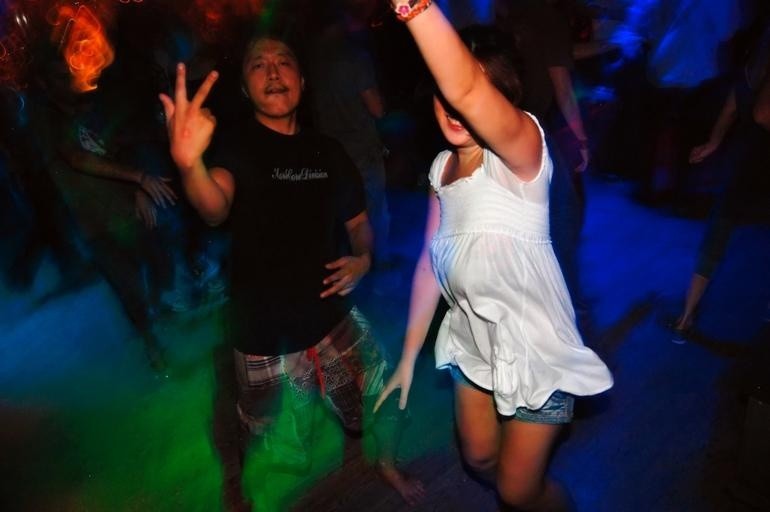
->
xmin=156 ymin=25 xmax=430 ymax=512
xmin=1 ymin=2 xmax=769 ymax=372
xmin=371 ymin=1 xmax=619 ymax=510
